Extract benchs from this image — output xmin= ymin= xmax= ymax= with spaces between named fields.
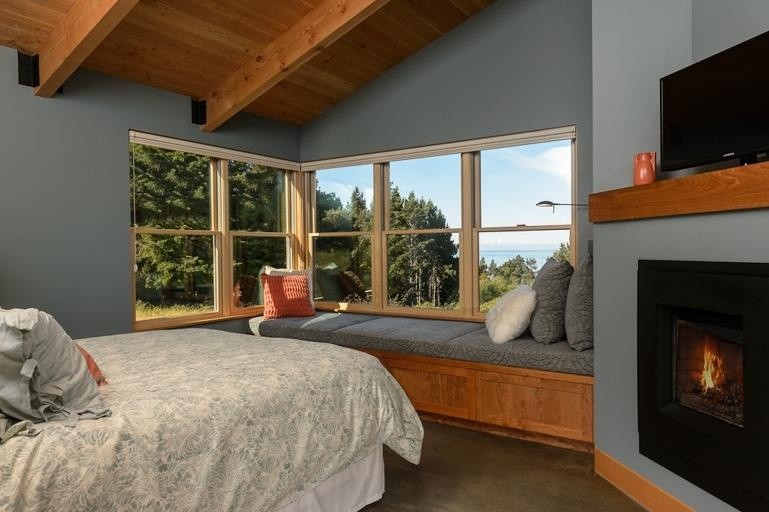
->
xmin=245 ymin=310 xmax=595 ymax=455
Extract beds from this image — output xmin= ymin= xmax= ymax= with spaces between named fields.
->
xmin=0 ymin=326 xmax=425 ymax=512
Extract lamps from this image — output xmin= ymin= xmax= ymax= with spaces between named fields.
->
xmin=535 ymin=200 xmax=589 ymax=213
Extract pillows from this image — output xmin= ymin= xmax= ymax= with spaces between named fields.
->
xmin=564 ymin=253 xmax=594 ymax=352
xmin=339 ymin=270 xmax=366 ymax=301
xmin=72 ymin=340 xmax=109 ymax=386
xmin=484 ymin=284 xmax=537 ymax=345
xmin=530 ymin=254 xmax=575 ymax=345
xmin=265 ymin=265 xmax=316 ymax=309
xmin=259 ymin=273 xmax=316 ymax=321
xmin=257 ymin=265 xmax=265 ymax=305
xmin=315 ymin=262 xmax=346 ymax=303
xmin=0 ymin=308 xmax=112 ymax=423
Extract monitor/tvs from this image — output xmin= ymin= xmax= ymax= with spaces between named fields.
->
xmin=659 ymin=30 xmax=769 ymax=172
